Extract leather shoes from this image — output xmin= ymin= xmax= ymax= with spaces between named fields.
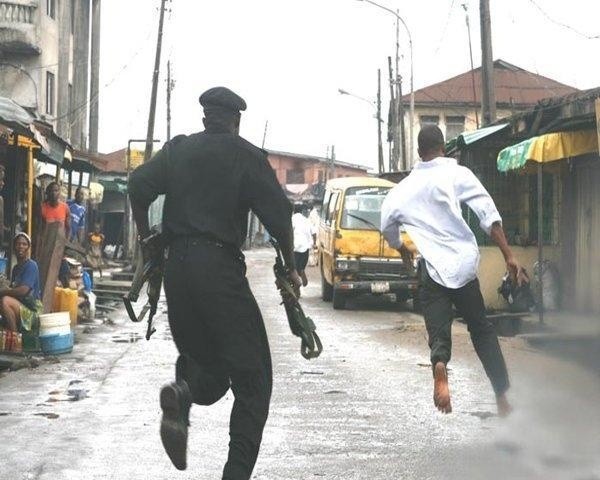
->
xmin=160 ymin=380 xmax=192 ymax=471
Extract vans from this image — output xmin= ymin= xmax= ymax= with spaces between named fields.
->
xmin=317 ymin=176 xmax=422 ymax=314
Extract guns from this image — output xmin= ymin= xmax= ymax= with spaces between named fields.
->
xmin=268 ymin=237 xmax=322 ymax=360
xmin=497 ymin=267 xmax=535 ymax=313
xmin=128 ymin=224 xmax=166 ymax=340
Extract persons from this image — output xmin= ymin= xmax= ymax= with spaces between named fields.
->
xmin=291 ymin=202 xmax=320 ymax=287
xmin=40 ymin=181 xmax=72 ymax=288
xmin=125 ymin=85 xmax=302 ymax=480
xmin=88 ymin=222 xmax=106 ymax=281
xmin=63 ymin=187 xmax=89 ymax=242
xmin=379 ymin=124 xmax=531 ymax=419
xmin=0 ymin=231 xmax=45 ymax=332
xmin=0 ymin=163 xmax=11 ymax=258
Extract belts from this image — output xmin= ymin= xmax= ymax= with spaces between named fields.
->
xmin=171 ymin=236 xmax=243 ymax=260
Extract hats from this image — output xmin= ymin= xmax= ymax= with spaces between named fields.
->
xmin=199 ymin=87 xmax=248 ymax=111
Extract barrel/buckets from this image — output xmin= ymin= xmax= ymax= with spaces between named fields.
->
xmin=36 ymin=310 xmax=72 ymax=353
